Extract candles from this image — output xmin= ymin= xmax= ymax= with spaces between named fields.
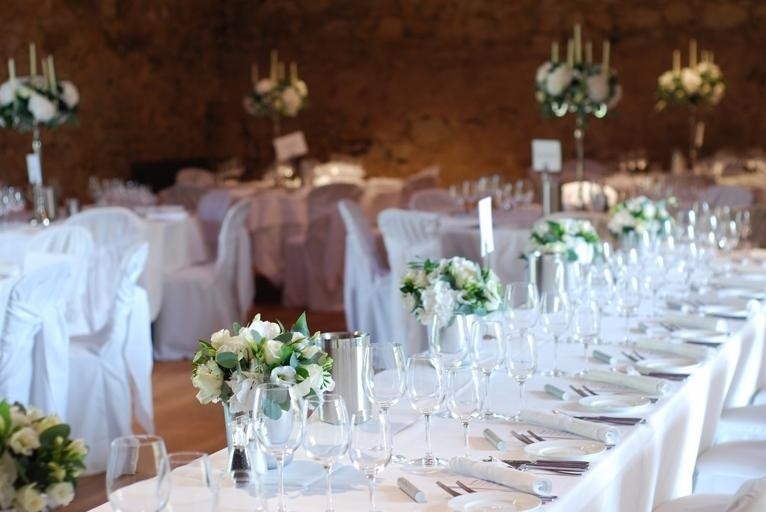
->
xmin=6 ymin=42 xmax=58 ymax=99
xmin=671 ymin=37 xmax=714 ymax=77
xmin=550 ymin=22 xmax=611 ymax=72
xmin=248 ymin=47 xmax=297 ymax=83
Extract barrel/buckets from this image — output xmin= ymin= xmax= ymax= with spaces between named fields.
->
xmin=318 ymin=333 xmax=373 ymax=424
xmin=525 ymin=252 xmax=579 ymax=307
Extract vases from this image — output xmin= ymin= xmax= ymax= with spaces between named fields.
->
xmin=24 ymin=123 xmax=60 ymax=225
xmin=685 ymin=95 xmax=700 ymax=175
xmin=570 ymin=108 xmax=590 ymax=184
xmin=268 ymin=111 xmax=291 ymax=191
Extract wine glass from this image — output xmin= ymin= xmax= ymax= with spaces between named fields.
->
xmin=253 ymin=385 xmax=393 ymax=510
xmin=231 ymin=153 xmax=370 ymax=191
xmin=0 ymin=172 xmax=152 ymax=236
xmin=447 ymin=148 xmax=727 ymax=215
xmin=396 ymin=199 xmax=754 ymax=474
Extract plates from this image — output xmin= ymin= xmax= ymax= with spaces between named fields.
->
xmin=447 ymin=490 xmax=542 ymax=512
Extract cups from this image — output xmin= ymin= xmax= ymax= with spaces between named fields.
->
xmin=106 ymin=431 xmax=212 ymax=511
xmin=320 ymin=331 xmax=371 ymax=410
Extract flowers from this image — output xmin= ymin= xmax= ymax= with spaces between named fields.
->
xmin=534 ymin=62 xmax=622 ymax=120
xmin=0 ymin=74 xmax=80 ymax=132
xmin=243 ymin=78 xmax=308 ymax=119
xmin=656 ymin=60 xmax=725 ymax=109
